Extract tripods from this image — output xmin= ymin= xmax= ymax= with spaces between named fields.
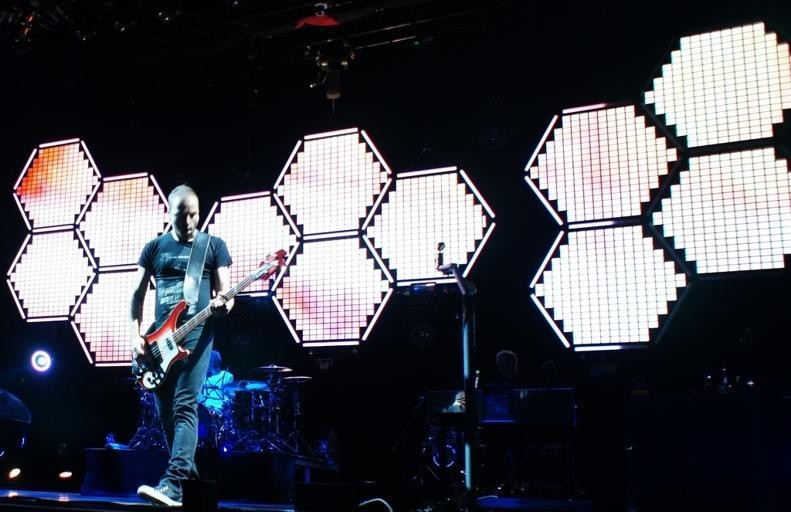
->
xmin=284 ymin=385 xmax=317 ymax=457
xmin=254 ymin=376 xmax=297 ymax=454
xmin=231 ymin=392 xmax=284 ymax=452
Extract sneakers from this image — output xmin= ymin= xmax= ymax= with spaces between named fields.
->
xmin=137 ymin=484 xmax=183 ymax=508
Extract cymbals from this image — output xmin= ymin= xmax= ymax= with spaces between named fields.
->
xmin=220 ymin=379 xmax=269 ymax=395
xmin=249 ymin=364 xmax=294 ymax=374
xmin=281 ymin=376 xmax=313 ymax=382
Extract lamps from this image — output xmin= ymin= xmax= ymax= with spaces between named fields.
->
xmin=311 ymin=27 xmax=357 ymax=106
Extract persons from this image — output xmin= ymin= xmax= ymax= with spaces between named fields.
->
xmin=129 ymin=184 xmax=236 ymax=507
xmin=484 ymin=349 xmax=529 ymax=497
xmin=199 ymin=350 xmax=235 ymax=417
xmin=442 ymin=391 xmax=466 ymax=413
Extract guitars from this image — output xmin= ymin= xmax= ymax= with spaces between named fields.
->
xmin=131 ymin=262 xmax=278 ymax=391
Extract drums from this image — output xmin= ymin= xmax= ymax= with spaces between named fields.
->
xmin=271 ymin=387 xmax=302 ymax=415
xmin=198 ymin=403 xmax=226 ymax=449
xmin=233 ymin=392 xmax=274 ymax=426
